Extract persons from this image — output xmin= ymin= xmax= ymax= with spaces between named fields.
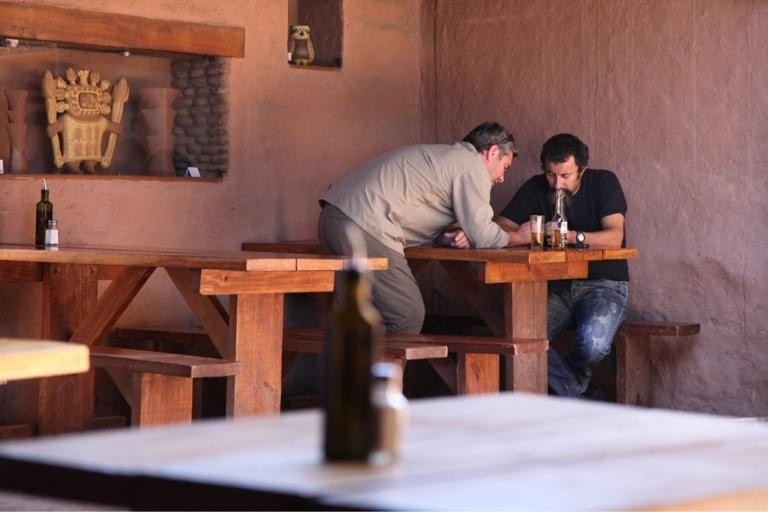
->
xmin=318 ymin=122 xmax=532 ymax=371
xmin=495 ymin=132 xmax=631 ymax=398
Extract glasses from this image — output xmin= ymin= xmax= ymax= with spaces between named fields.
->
xmin=496 ymin=134 xmax=514 ymax=144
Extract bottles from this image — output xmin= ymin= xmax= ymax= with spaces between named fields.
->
xmin=45 ymin=219 xmax=59 ymax=250
xmin=35 ymin=189 xmax=53 ymax=250
xmin=551 ymin=189 xmax=568 ymax=250
xmin=320 ymin=266 xmax=379 ymax=465
xmin=369 ymin=362 xmax=412 ymax=471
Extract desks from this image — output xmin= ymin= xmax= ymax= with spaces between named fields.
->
xmin=0 ymin=240 xmax=768 ymax=512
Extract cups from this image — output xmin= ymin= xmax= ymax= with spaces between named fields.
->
xmin=530 ymin=215 xmax=545 ymax=249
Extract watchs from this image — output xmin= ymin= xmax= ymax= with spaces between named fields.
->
xmin=575 ymin=231 xmax=585 ymax=245
